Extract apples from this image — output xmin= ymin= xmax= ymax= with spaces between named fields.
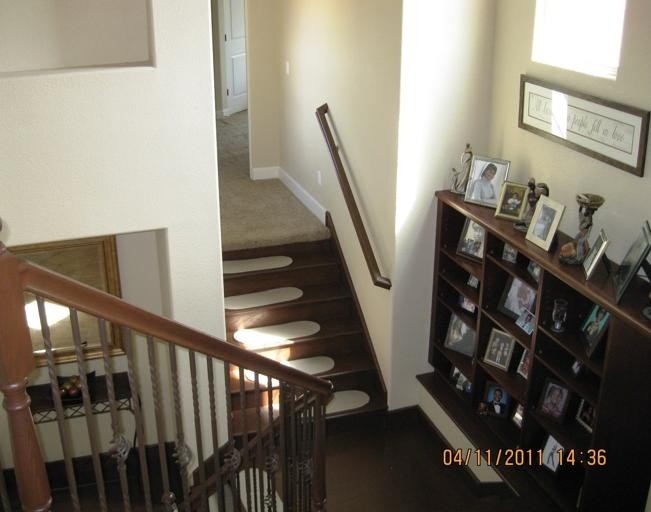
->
xmin=59 ymin=374 xmax=81 ymax=397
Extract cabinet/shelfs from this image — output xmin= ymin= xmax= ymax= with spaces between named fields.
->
xmin=415 ymin=189 xmax=650 ymax=512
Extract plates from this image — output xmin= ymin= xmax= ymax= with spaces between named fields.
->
xmin=46 ymin=371 xmax=95 ymax=402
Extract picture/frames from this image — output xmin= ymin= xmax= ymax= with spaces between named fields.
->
xmin=467 ymin=274 xmax=479 ymax=289
xmin=464 ymin=156 xmax=511 ymax=208
xmin=536 ymin=376 xmax=572 ymax=425
xmin=518 ymin=350 xmax=531 ymax=379
xmin=583 ymin=228 xmax=611 ymax=281
xmin=576 ymin=399 xmax=593 ymax=434
xmin=525 ymin=194 xmax=566 ymax=251
xmin=497 ymin=277 xmax=538 ymax=320
xmin=456 ymin=219 xmax=486 ymax=261
xmin=612 ymin=219 xmax=650 ymax=305
xmin=517 ymin=73 xmax=650 ymax=178
xmin=483 ymin=380 xmax=511 ymax=419
xmin=7 ymin=236 xmax=128 ymax=368
xmin=512 ymin=403 xmax=524 ymax=429
xmin=580 ymin=305 xmax=611 ymax=358
xmin=541 ymin=434 xmax=565 ymax=473
xmin=445 ymin=314 xmax=476 ymax=357
xmin=484 ymin=327 xmax=515 ymax=370
xmin=502 ymin=242 xmax=519 ymax=264
xmin=515 ymin=309 xmax=537 ymax=335
xmin=494 ymin=182 xmax=531 ymax=223
xmin=527 ymin=262 xmax=539 ymax=284
xmin=449 ymin=365 xmax=473 ymax=393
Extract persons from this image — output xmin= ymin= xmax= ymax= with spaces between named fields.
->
xmin=507 ymin=193 xmax=521 ymax=211
xmin=488 ymin=337 xmax=510 ymax=365
xmin=517 ymin=313 xmax=532 ymax=333
xmin=488 ymin=388 xmax=506 ymax=416
xmin=470 ymin=162 xmax=499 ymax=203
xmin=543 ymin=387 xmax=563 ymax=417
xmin=546 ymin=443 xmax=558 ymax=469
xmin=585 ymin=306 xmax=608 ymax=340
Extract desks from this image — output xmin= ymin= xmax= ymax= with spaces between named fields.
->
xmin=26 ymin=372 xmax=141 ymax=454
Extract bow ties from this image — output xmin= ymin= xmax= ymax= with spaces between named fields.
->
xmin=495 ymin=402 xmax=500 ymax=405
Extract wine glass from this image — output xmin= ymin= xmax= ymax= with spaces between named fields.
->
xmin=550 ymin=298 xmax=568 ymax=333
